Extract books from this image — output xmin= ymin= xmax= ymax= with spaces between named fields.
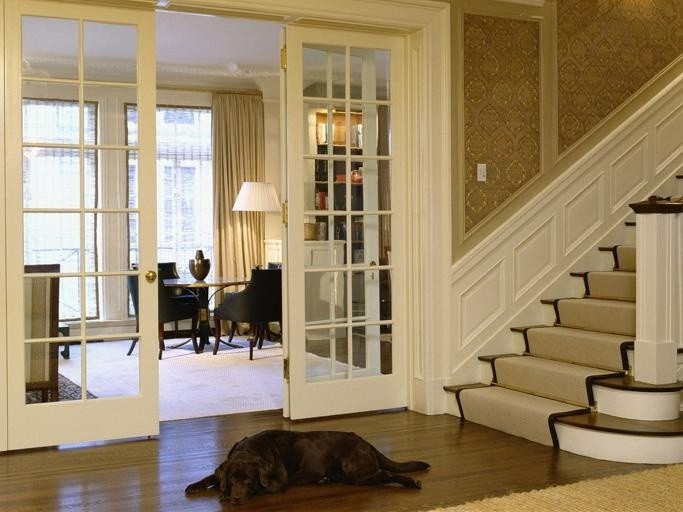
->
xmin=314 ymin=121 xmax=364 ymax=263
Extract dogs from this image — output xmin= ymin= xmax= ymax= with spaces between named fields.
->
xmin=184 ymin=430 xmax=431 ymax=512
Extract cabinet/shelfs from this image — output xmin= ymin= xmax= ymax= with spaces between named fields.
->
xmin=304 ymin=83 xmax=390 ymax=334
xmin=264 ymin=240 xmax=346 ymax=342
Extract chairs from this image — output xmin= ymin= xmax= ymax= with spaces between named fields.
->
xmin=126 ymin=262 xmax=281 ymax=359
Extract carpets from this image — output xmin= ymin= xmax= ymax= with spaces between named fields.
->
xmin=58 ymin=335 xmax=360 ymax=422
xmin=456 ymin=196 xmax=683 ymax=448
xmin=427 ymin=462 xmax=683 ymax=512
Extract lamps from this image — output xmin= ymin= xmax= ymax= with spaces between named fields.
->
xmin=232 ymin=182 xmax=287 ymax=228
